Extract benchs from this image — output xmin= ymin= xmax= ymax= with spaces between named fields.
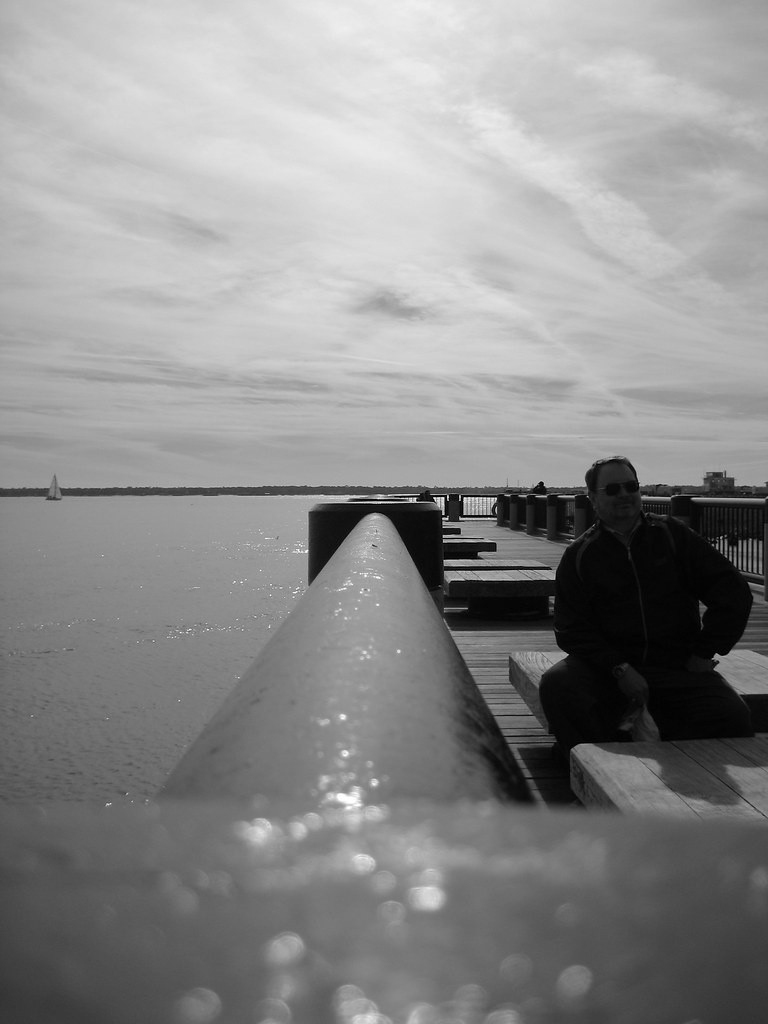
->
xmin=442 ymin=525 xmax=558 ymax=626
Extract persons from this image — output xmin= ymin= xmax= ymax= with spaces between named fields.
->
xmin=538 ymin=456 xmax=755 ymax=745
xmin=533 ymin=481 xmax=546 ymax=493
xmin=416 ymin=490 xmax=436 ymax=504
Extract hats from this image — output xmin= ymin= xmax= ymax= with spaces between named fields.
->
xmin=538 ymin=481 xmax=543 ymax=485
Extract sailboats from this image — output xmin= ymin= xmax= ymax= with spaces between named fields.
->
xmin=44 ymin=473 xmax=63 ymax=500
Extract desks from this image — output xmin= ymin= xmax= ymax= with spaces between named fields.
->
xmin=507 ymin=645 xmax=768 ymax=822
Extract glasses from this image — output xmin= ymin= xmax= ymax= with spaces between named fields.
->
xmin=596 ymin=480 xmax=640 ymax=497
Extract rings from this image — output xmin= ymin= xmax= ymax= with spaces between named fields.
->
xmin=630 ymin=697 xmax=636 ymax=702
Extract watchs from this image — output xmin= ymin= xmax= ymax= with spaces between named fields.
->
xmin=612 ymin=662 xmax=631 ymax=679
